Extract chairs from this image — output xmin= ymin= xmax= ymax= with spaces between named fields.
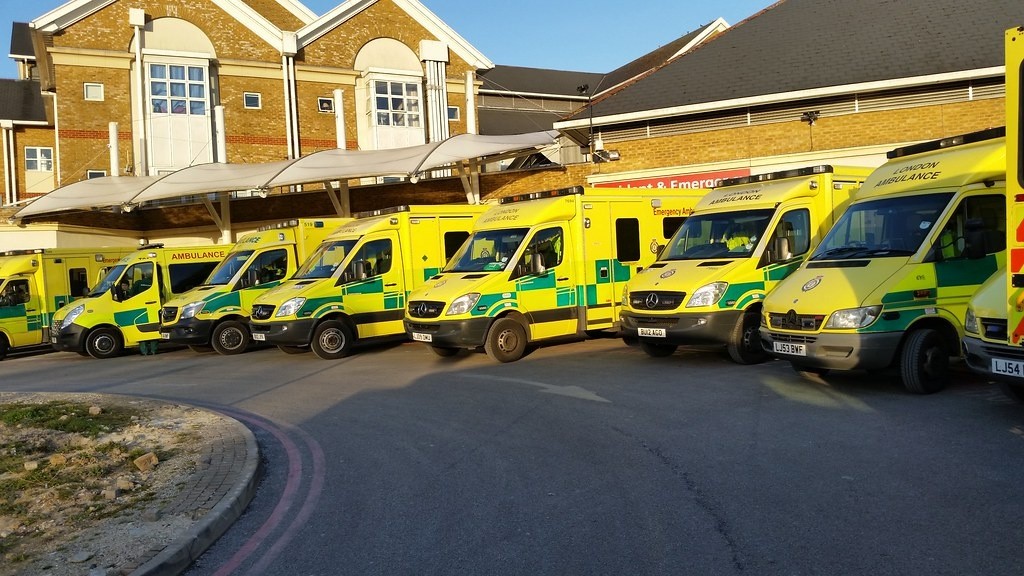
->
xmin=759 ymin=219 xmax=792 ymax=259
xmin=887 ymin=213 xmax=918 ymax=251
xmin=259 ymin=267 xmax=278 ymax=284
xmin=375 ymin=248 xmax=391 ymax=273
xmin=535 ymin=241 xmax=557 ymax=268
xmin=961 ymin=219 xmax=989 ymax=259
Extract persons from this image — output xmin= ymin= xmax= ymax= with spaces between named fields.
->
xmin=129 ymin=269 xmax=159 ymax=356
xmin=118 ymin=280 xmax=130 ymax=302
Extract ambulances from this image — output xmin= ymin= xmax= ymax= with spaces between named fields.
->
xmin=249 ymin=204 xmax=496 ymax=360
xmin=0 ymin=247 xmax=141 ymax=362
xmin=617 ymin=165 xmax=877 ymax=365
xmin=400 ymin=183 xmax=717 ymax=363
xmin=758 ymin=122 xmax=1006 ymax=393
xmin=51 ymin=242 xmax=234 ymax=359
xmin=158 ymin=217 xmax=357 ymax=355
xmin=961 ymin=260 xmax=1024 ymax=414
